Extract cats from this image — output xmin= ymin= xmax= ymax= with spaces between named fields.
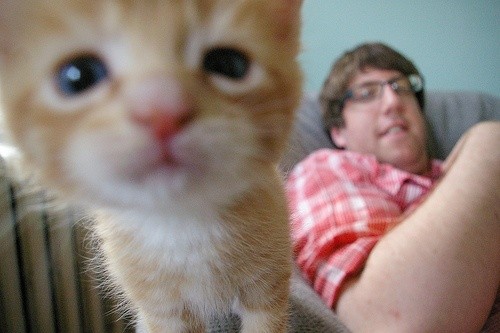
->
xmin=0 ymin=0 xmax=305 ymax=333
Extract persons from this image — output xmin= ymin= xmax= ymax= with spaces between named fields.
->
xmin=285 ymin=42 xmax=500 ymax=333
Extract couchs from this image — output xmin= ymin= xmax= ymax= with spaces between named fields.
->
xmin=0 ymin=91 xmax=500 ymax=333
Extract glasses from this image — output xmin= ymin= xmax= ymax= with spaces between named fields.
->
xmin=339 ymin=74 xmax=424 ymax=104
